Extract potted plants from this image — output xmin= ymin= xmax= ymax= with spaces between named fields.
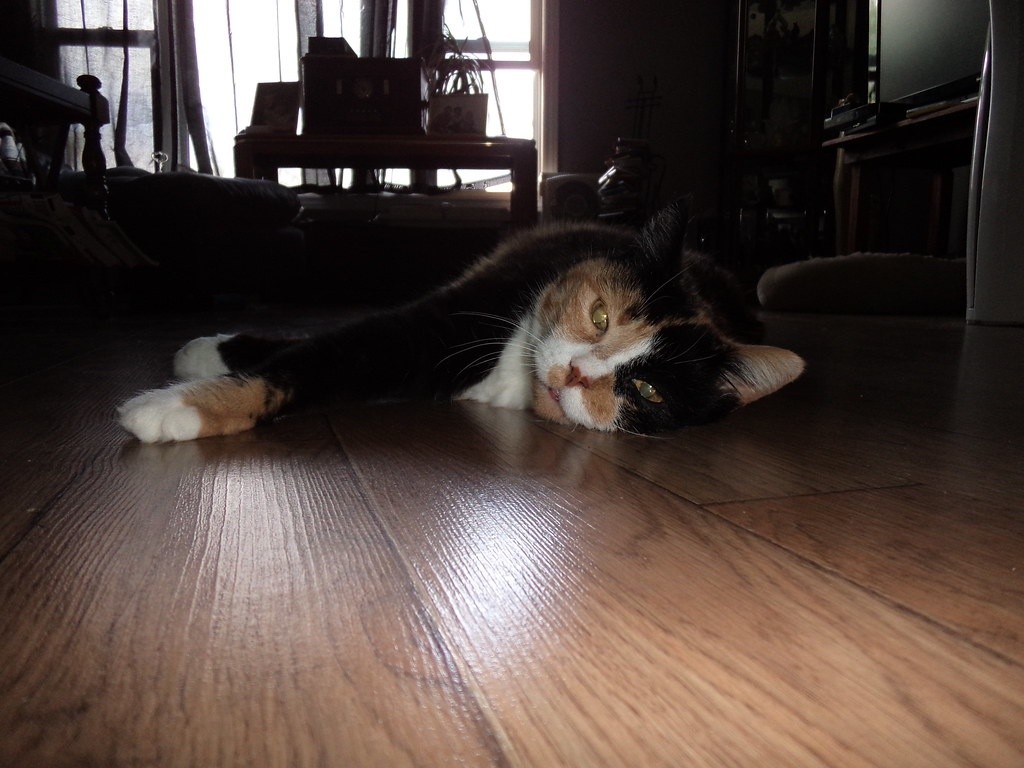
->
xmin=419 ymin=24 xmax=488 ymax=137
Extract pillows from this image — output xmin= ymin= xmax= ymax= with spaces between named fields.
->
xmin=108 ymin=165 xmax=302 ymax=225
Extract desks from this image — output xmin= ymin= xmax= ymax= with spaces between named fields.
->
xmin=820 ymin=97 xmax=979 ymax=256
xmin=226 ymin=131 xmax=539 ymax=220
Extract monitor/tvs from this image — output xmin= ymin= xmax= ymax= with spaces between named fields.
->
xmin=868 ymin=0 xmax=990 ymax=117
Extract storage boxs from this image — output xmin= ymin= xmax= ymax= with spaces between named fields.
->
xmin=300 ymin=54 xmax=423 ymax=133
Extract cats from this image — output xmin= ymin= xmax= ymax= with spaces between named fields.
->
xmin=115 ymin=200 xmax=810 ymax=444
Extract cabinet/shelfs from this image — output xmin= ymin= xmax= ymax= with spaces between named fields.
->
xmin=0 ymin=58 xmax=119 ymax=308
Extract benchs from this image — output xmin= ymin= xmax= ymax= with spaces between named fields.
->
xmin=65 ymin=189 xmax=520 ymax=300
